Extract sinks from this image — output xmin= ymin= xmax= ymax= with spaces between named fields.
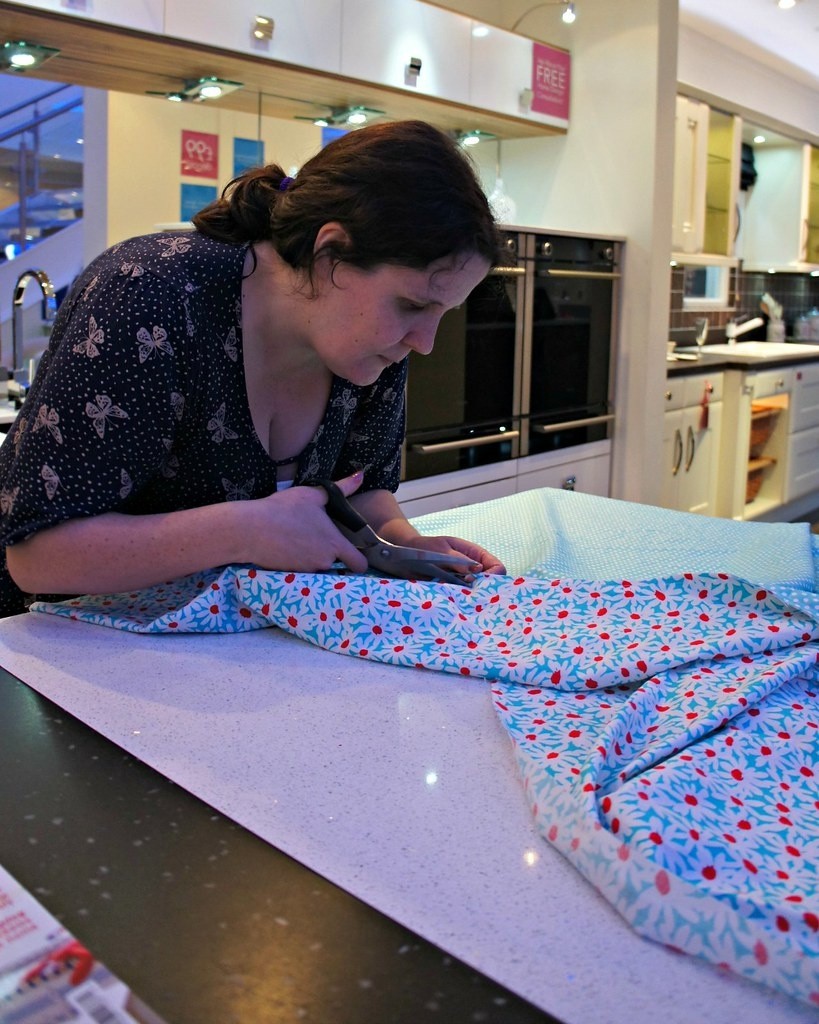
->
xmin=691 ymin=341 xmax=818 ymax=357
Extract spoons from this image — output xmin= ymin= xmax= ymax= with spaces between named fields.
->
xmin=760 ymin=303 xmax=770 ymax=315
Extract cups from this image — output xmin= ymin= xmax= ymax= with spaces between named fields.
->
xmin=766 ymin=319 xmax=786 ymax=343
xmin=667 ymin=341 xmax=677 ymax=356
xmin=793 ymin=314 xmax=819 ymax=340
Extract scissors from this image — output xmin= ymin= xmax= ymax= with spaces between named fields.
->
xmin=299 ymin=478 xmax=479 ymax=588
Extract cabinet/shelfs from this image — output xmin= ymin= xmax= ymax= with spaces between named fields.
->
xmin=666 ymin=371 xmax=722 ymax=517
xmin=741 ymin=142 xmax=819 ymax=273
xmin=397 ymin=439 xmax=612 ymax=516
xmin=671 ymin=92 xmax=742 ymax=267
xmin=723 ymin=369 xmax=819 ymax=523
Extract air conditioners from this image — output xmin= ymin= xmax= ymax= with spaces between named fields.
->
xmin=0 ymin=41 xmax=62 ymax=72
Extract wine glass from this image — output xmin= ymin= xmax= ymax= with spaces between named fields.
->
xmin=694 ymin=316 xmax=709 ymax=359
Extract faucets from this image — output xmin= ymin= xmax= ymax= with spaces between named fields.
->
xmin=725 ymin=313 xmax=764 ymax=346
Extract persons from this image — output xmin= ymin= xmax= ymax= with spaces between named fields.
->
xmin=0 ymin=120 xmax=511 ymax=621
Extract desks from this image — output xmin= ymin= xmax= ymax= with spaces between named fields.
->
xmin=0 ymin=487 xmax=819 ymax=1024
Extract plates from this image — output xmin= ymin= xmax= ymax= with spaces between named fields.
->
xmin=676 ymin=354 xmax=698 ymax=361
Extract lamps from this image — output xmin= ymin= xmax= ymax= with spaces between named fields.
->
xmin=455 ymin=128 xmax=496 ymax=150
xmin=146 ymin=76 xmax=244 ymax=102
xmin=293 ymin=106 xmax=387 ymax=126
